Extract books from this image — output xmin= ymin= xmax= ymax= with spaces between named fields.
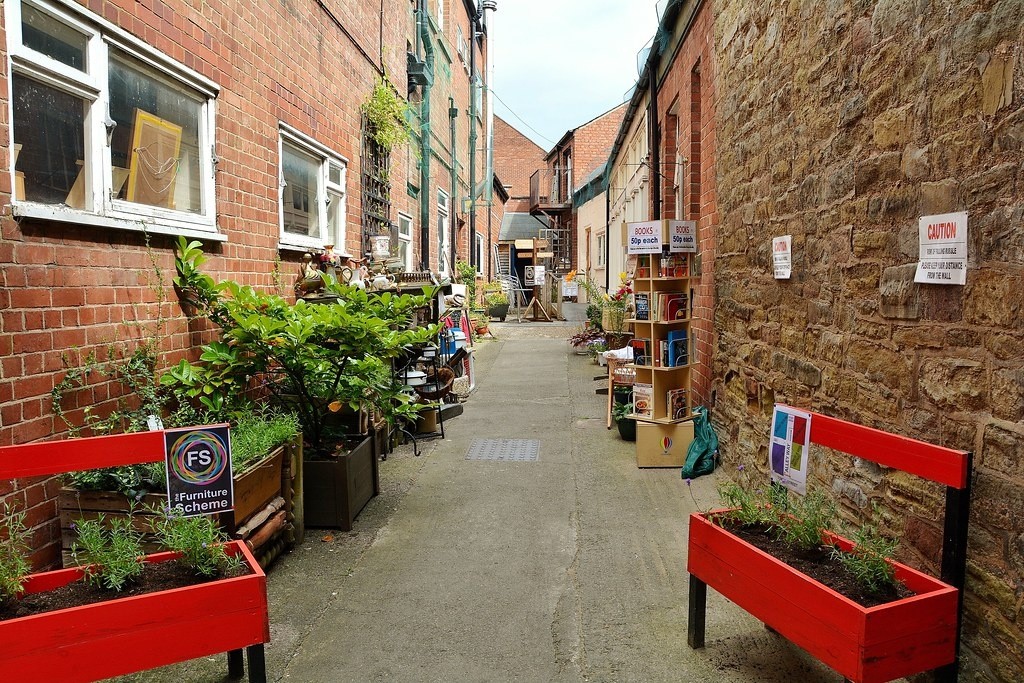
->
xmin=633 ymin=252 xmax=687 ymax=419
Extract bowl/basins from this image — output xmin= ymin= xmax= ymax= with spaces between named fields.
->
xmin=401 ymin=370 xmax=428 ymax=385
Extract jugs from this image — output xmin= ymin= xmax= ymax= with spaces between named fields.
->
xmin=297 ymin=244 xmax=405 ymax=297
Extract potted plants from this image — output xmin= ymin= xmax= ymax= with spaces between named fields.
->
xmin=0 ymin=233 xmax=969 ymax=683
